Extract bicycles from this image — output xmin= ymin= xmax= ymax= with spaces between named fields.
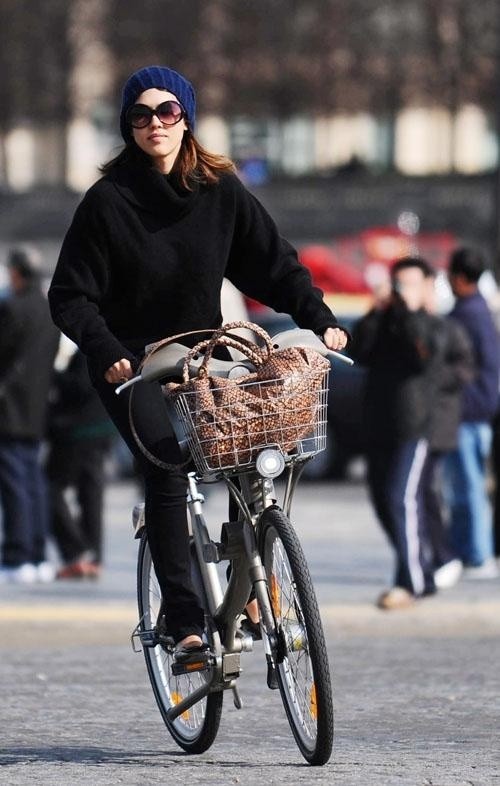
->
xmin=115 ymin=328 xmax=355 ymax=765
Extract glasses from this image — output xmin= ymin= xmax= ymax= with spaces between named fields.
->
xmin=125 ymin=101 xmax=186 ymax=129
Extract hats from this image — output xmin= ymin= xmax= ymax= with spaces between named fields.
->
xmin=121 ymin=66 xmax=196 ymax=129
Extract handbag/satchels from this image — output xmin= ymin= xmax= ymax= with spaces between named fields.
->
xmin=170 ymin=321 xmax=331 ymax=467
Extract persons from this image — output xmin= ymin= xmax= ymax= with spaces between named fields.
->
xmin=48 ymin=66 xmax=354 ymax=657
xmin=0 ymin=240 xmax=61 ymax=584
xmin=349 ymin=255 xmax=474 ymax=609
xmin=436 ymin=245 xmax=499 ymax=581
xmin=41 ymin=347 xmax=117 ymax=580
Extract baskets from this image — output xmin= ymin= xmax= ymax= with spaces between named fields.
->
xmin=173 ymin=368 xmax=331 ymax=476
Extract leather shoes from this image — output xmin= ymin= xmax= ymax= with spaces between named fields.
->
xmin=175 ymin=635 xmax=209 ymax=664
xmin=227 ymin=565 xmax=262 ymax=639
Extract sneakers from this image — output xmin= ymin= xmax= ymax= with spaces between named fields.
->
xmin=433 ymin=556 xmax=499 ymax=589
xmin=0 ymin=560 xmax=102 ymax=584
xmin=379 ymin=586 xmax=415 ymax=609
